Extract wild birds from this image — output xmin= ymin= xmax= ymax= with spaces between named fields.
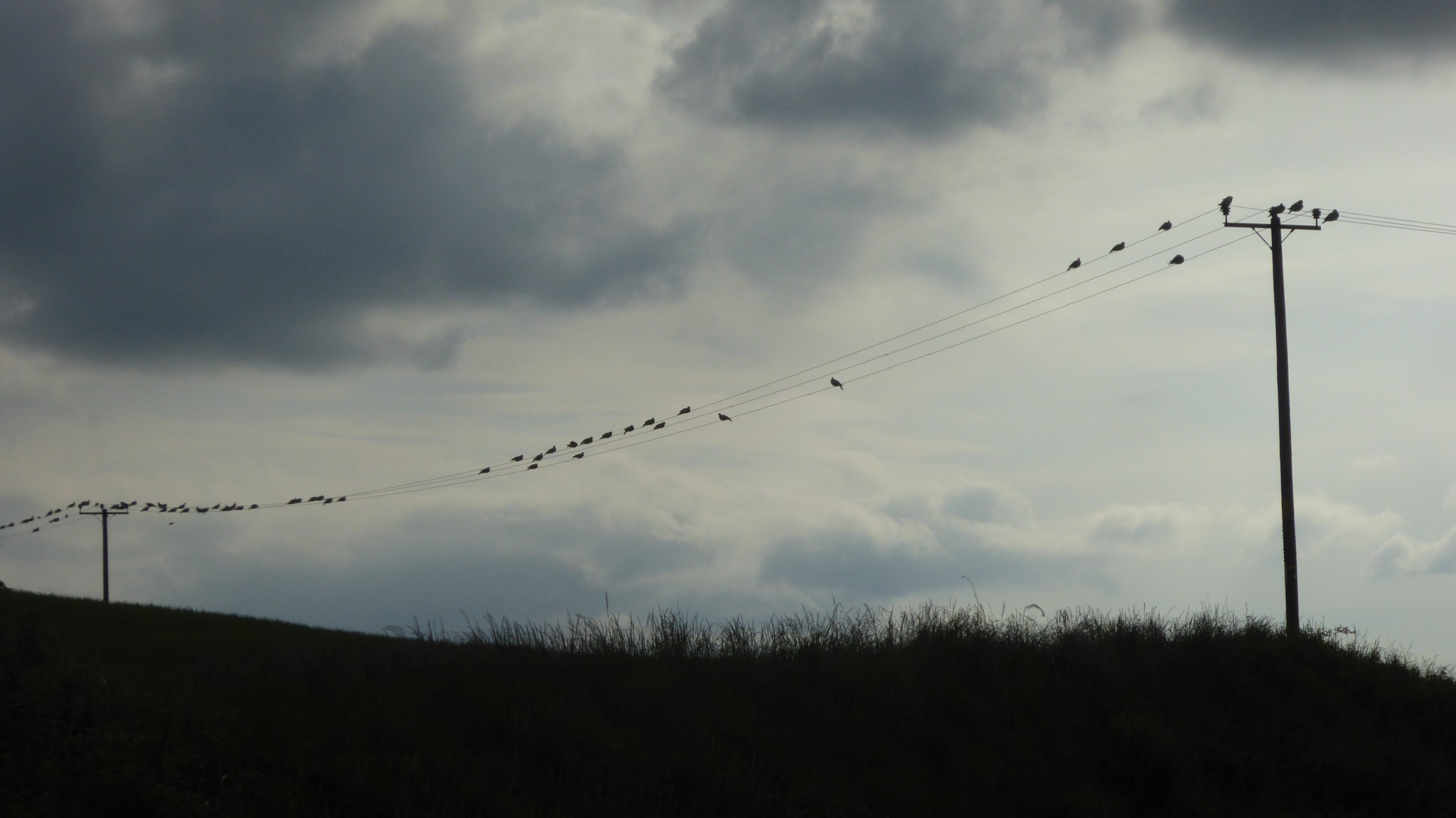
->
xmin=6 ymin=496 xmax=346 ymax=532
xmin=1068 ymin=196 xmax=1339 ymax=273
xmin=478 ymin=376 xmax=844 ymax=474
